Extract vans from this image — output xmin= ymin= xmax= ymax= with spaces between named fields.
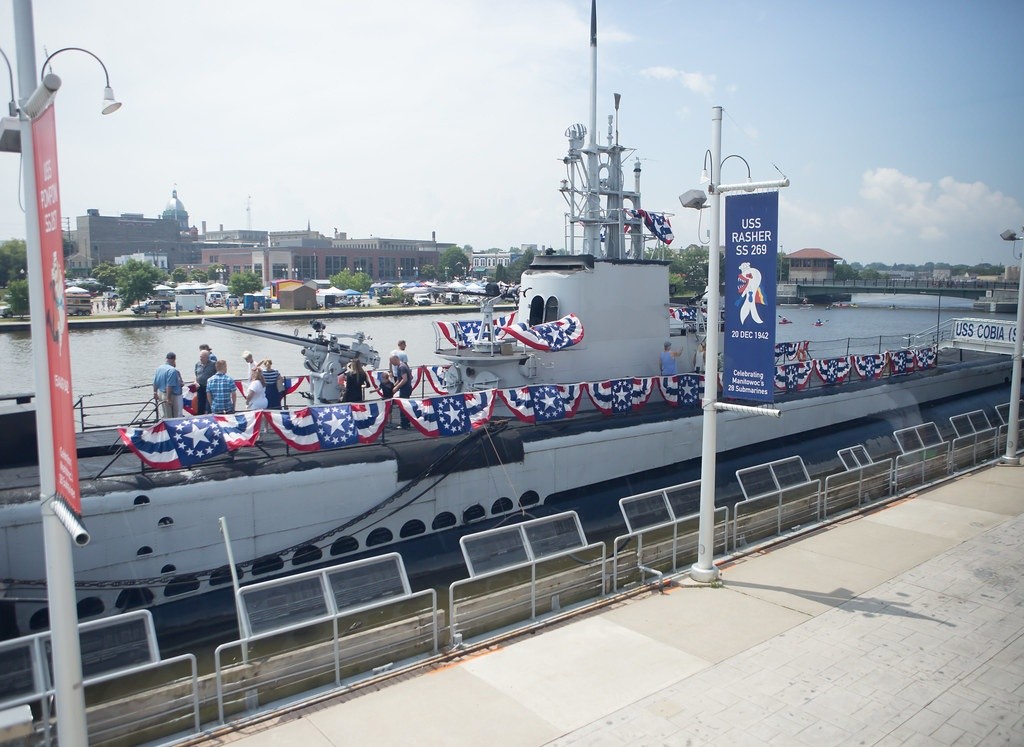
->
xmin=206 ymin=292 xmax=222 ymax=305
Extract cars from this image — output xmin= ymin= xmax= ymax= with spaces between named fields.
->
xmin=227 ymin=293 xmax=239 ymax=305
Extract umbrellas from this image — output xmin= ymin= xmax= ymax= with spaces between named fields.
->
xmin=598 ymin=208 xmax=674 ymax=245
xmin=322 ymin=281 xmax=495 ymax=295
xmin=152 ymin=281 xmax=235 ymax=294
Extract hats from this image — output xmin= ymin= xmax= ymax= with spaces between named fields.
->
xmin=166 ymin=352 xmax=175 ymax=360
xmin=199 ymin=345 xmax=212 ymax=351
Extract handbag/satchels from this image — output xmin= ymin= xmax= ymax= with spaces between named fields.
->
xmin=276 ymin=379 xmax=285 ymax=392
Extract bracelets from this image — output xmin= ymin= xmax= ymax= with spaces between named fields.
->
xmin=153 ymin=392 xmax=157 ymax=395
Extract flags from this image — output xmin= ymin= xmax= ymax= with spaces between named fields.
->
xmin=180 ymin=305 xmax=936 ymax=451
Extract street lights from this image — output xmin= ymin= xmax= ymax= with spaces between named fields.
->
xmin=1 ymin=46 xmax=122 ymax=747
xmin=693 ymin=147 xmax=758 ymax=579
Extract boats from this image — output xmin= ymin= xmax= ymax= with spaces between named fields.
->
xmin=814 ymin=321 xmax=823 ymax=327
xmin=779 ymin=321 xmax=791 ymax=324
xmin=0 ymin=1 xmax=1022 ymax=652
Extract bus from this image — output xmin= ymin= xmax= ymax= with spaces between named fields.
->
xmin=66 ymin=294 xmax=92 ymax=316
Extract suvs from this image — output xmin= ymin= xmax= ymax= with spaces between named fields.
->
xmin=414 ymin=294 xmax=432 ymax=306
xmin=131 ymin=300 xmax=171 ymax=314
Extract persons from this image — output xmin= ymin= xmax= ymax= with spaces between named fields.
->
xmin=96 ymin=295 xmax=365 ymax=312
xmin=153 ymin=339 xmax=707 ymax=418
